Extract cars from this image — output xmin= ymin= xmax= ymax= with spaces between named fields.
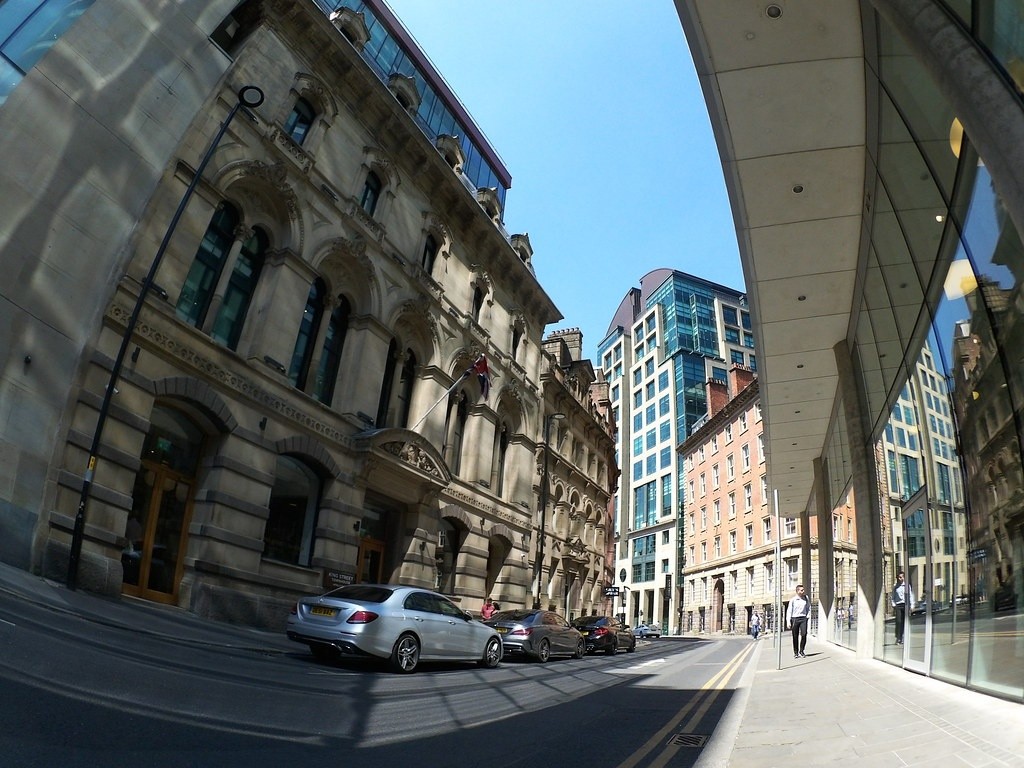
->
xmin=571 ymin=616 xmax=635 ymax=656
xmin=481 ymin=609 xmax=586 ymax=662
xmin=285 ymin=582 xmax=505 ymax=674
xmin=990 ymin=565 xmax=1024 ymax=615
xmin=633 ymin=624 xmax=661 ymax=638
xmin=950 ymin=595 xmax=968 ymax=606
xmin=912 ymin=601 xmax=938 ymax=615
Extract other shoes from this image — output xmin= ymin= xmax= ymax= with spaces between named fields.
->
xmin=895 ymin=638 xmax=904 ymax=645
xmin=799 ymin=651 xmax=806 ymax=658
xmin=794 ymin=653 xmax=798 ymax=657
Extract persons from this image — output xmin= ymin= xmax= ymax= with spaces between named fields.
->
xmin=890 ymin=572 xmax=915 ymax=645
xmin=786 ymin=584 xmax=812 ymax=658
xmin=837 ymin=602 xmax=853 ymax=630
xmin=642 ymin=620 xmax=647 ymax=626
xmin=481 ymin=598 xmax=500 ymax=622
xmin=751 ymin=611 xmax=760 ymax=641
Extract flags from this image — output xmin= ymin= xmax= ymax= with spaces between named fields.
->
xmin=466 ymin=354 xmax=492 ymax=401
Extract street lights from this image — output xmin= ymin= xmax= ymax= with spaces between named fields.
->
xmin=537 ymin=413 xmax=566 ymax=609
xmin=68 ymin=85 xmax=266 ymax=590
